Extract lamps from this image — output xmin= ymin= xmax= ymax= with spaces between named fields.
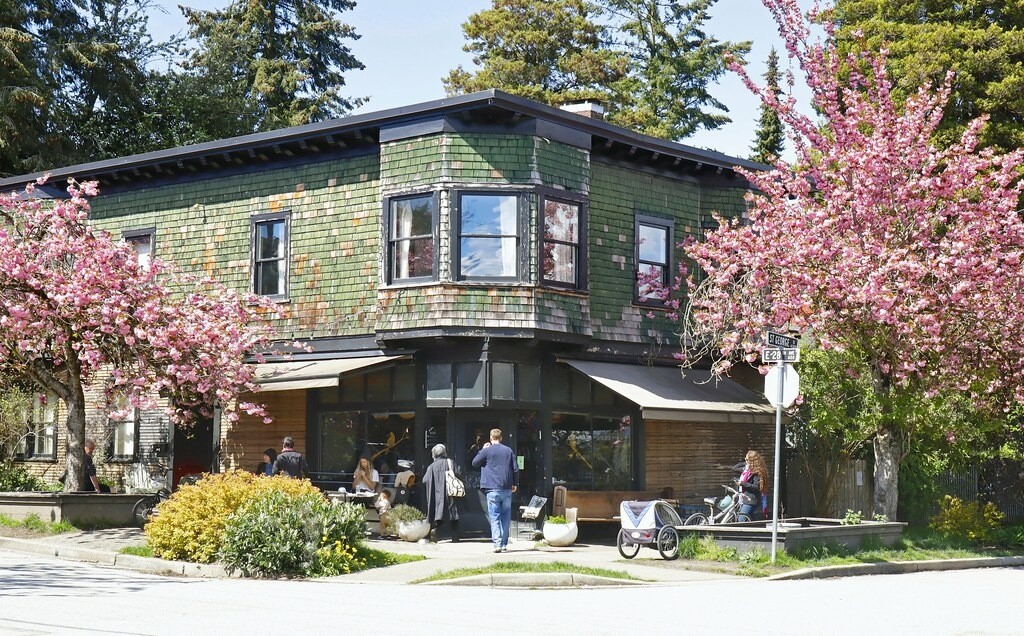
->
xmin=192 ymin=203 xmax=206 ymax=211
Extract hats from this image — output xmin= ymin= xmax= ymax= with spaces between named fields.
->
xmin=359 ymin=452 xmax=371 ymax=461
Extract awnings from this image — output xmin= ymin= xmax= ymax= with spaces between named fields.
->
xmin=158 ymin=353 xmax=414 ymax=399
xmin=551 ymin=350 xmax=791 ymax=425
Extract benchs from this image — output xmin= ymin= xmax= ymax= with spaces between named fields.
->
xmin=554 ymin=485 xmax=674 ymax=526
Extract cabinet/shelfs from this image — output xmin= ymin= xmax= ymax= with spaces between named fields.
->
xmin=516 ymin=511 xmax=544 ymax=540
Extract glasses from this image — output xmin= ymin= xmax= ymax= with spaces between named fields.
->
xmin=745 ymin=458 xmax=748 ymax=460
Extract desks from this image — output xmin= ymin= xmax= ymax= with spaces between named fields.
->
xmin=327 ymin=493 xmax=378 ymax=504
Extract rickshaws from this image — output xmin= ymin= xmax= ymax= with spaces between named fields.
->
xmin=617 ymin=500 xmax=685 ymax=560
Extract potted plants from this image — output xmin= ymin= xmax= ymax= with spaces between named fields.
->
xmin=385 ymin=501 xmax=432 ymax=542
xmin=543 ymin=515 xmax=578 ymax=546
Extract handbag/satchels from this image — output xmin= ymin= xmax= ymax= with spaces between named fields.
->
xmin=58 ymin=472 xmax=66 ymax=485
xmin=445 ymin=459 xmax=465 ymax=497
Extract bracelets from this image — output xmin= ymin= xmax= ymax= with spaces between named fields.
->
xmin=353 ymin=476 xmax=356 ymax=478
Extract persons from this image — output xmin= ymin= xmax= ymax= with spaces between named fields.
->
xmin=658 ymin=487 xmax=683 ymax=517
xmin=713 ymin=450 xmax=768 ymax=523
xmin=422 ymin=444 xmax=464 ymax=542
xmin=82 ymin=440 xmax=110 ymax=494
xmin=471 ymin=428 xmax=520 ymax=552
xmin=394 ymin=457 xmax=419 ymax=507
xmin=352 ymin=453 xmax=379 ymax=508
xmin=272 ymin=436 xmax=309 ymax=479
xmin=466 ymin=433 xmax=485 ymax=472
xmin=255 ymin=447 xmax=280 ymax=477
xmin=375 ymin=489 xmax=392 ymax=540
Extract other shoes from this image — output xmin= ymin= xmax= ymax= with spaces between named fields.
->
xmin=501 ymin=545 xmax=506 ymax=550
xmin=387 ymin=535 xmax=391 ymax=539
xmin=452 ymin=534 xmax=459 ymax=542
xmin=493 ymin=547 xmax=501 ymax=553
xmin=377 ymin=536 xmax=384 ymax=539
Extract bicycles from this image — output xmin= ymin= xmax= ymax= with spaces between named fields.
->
xmin=132 ymin=475 xmax=201 ymax=531
xmin=684 ymin=484 xmax=753 ymax=526
